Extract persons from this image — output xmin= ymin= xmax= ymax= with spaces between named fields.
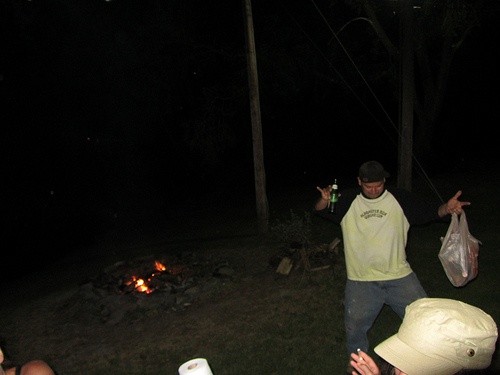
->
xmin=314 ymin=160 xmax=473 ymax=362
xmin=0 ymin=348 xmax=57 ymax=375
xmin=351 ymin=298 xmax=500 ymax=375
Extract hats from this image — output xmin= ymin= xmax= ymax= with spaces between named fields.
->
xmin=373 ymin=296 xmax=498 ymax=375
xmin=358 ymin=160 xmax=390 ymax=182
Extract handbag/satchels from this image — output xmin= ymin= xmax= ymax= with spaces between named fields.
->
xmin=438 ymin=208 xmax=482 ymax=287
